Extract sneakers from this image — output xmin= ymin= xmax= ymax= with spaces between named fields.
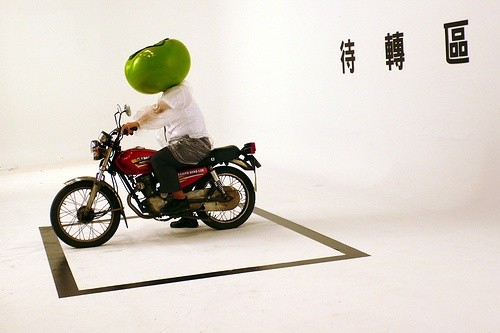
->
xmin=170 ymin=217 xmax=199 ymax=229
xmin=161 ymin=197 xmax=190 ymax=216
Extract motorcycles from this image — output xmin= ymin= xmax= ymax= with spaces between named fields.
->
xmin=49 ymin=103 xmax=261 ymax=249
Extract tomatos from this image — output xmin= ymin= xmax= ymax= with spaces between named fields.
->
xmin=124 ymin=38 xmax=191 ymax=95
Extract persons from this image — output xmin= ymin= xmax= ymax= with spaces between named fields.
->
xmin=120 ymin=84 xmax=213 ymax=228
